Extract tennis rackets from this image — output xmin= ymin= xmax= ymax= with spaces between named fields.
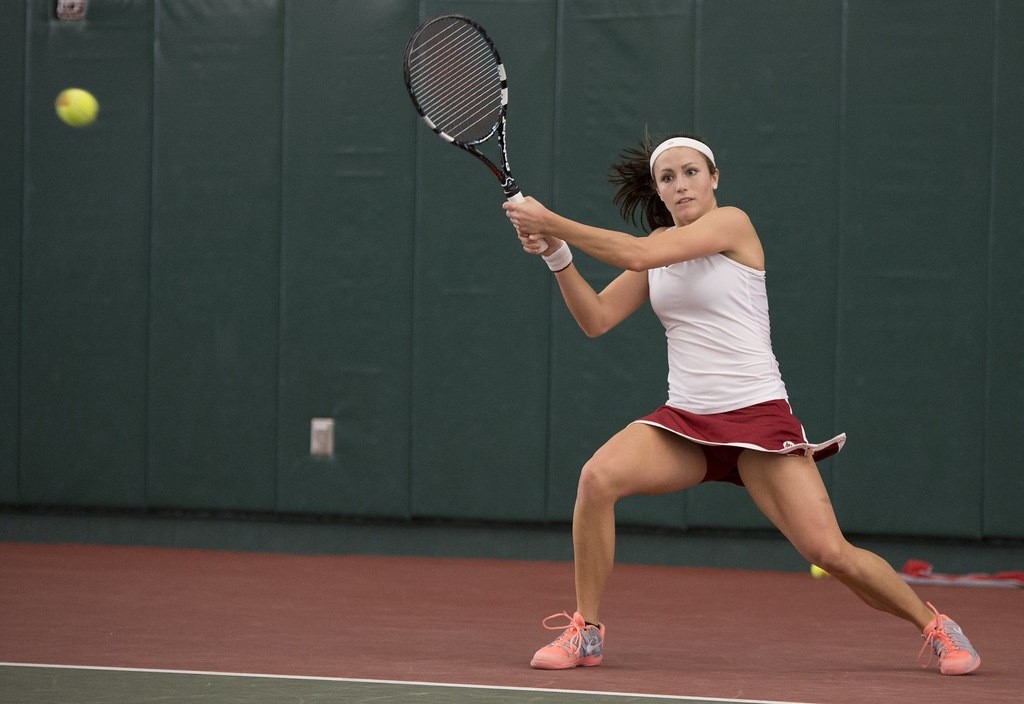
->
xmin=400 ymin=8 xmax=550 ymax=254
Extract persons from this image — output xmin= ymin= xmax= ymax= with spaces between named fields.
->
xmin=503 ymin=135 xmax=983 ymax=676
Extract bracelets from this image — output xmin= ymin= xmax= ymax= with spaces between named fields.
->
xmin=541 ymin=241 xmax=573 ymax=272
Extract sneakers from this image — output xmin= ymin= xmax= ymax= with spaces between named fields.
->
xmin=917 ymin=599 xmax=981 ymax=675
xmin=531 ymin=609 xmax=605 ymax=669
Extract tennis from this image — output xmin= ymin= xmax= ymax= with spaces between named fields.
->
xmin=54 ymin=87 xmax=100 ymax=129
